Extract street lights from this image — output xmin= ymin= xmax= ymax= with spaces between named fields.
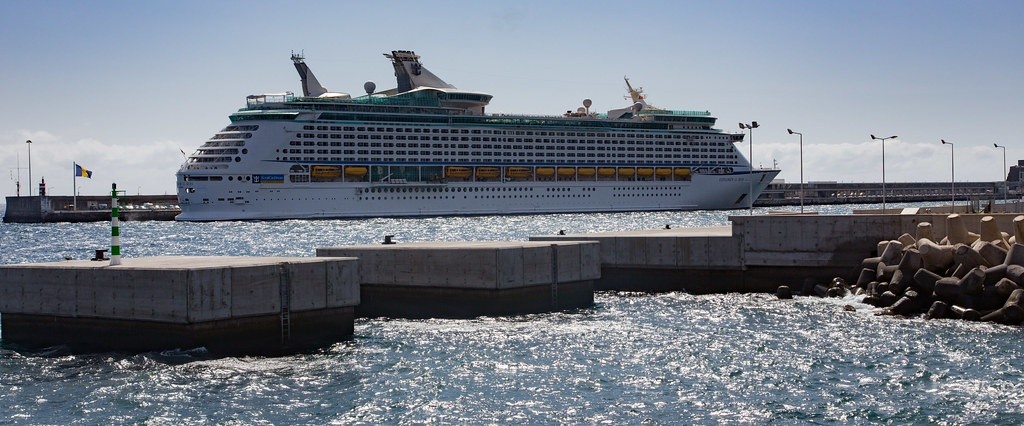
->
xmin=870 ymin=134 xmax=897 ymax=215
xmin=941 ymin=139 xmax=955 ymax=213
xmin=787 ymin=128 xmax=804 ymax=213
xmin=994 ymin=143 xmax=1007 ymax=213
xmin=26 ymin=140 xmax=32 ymax=196
xmin=739 ymin=121 xmax=760 ymax=215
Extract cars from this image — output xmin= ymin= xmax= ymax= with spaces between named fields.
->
xmin=118 ymin=203 xmax=180 ymax=210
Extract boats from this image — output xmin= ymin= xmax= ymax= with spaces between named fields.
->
xmin=174 ymin=49 xmax=781 ymax=223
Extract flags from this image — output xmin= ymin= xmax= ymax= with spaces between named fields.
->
xmin=76 ymin=164 xmax=92 ymax=179
